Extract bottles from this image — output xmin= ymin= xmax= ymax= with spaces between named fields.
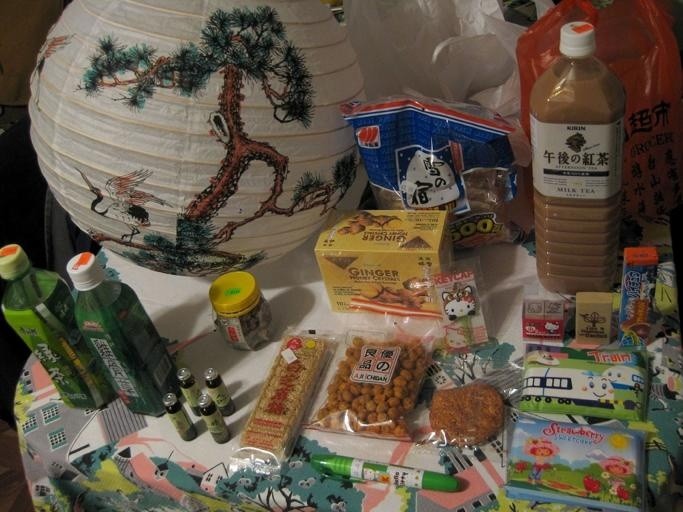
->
xmin=197 ymin=391 xmax=232 ymax=443
xmin=0 ymin=244 xmax=120 ymax=409
xmin=530 ymin=25 xmax=622 ymax=293
xmin=160 ymin=392 xmax=197 ymax=442
xmin=66 ymin=252 xmax=181 ymax=416
xmin=203 ymin=366 xmax=236 ymax=417
xmin=175 ymin=366 xmax=203 ymax=416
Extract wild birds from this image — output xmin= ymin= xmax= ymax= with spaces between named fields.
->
xmin=74 ymin=168 xmax=175 ymax=242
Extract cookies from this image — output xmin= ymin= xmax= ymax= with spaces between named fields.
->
xmin=429 ymin=383 xmax=504 ymax=447
xmin=239 ymin=336 xmax=327 ymax=460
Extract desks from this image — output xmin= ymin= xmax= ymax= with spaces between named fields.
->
xmin=13 ymin=241 xmax=683 ymax=511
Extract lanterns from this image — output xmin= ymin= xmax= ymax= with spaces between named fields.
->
xmin=28 ymin=0 xmax=370 ymax=279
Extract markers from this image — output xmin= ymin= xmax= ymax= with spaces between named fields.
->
xmin=309 ymin=453 xmax=460 ymax=496
